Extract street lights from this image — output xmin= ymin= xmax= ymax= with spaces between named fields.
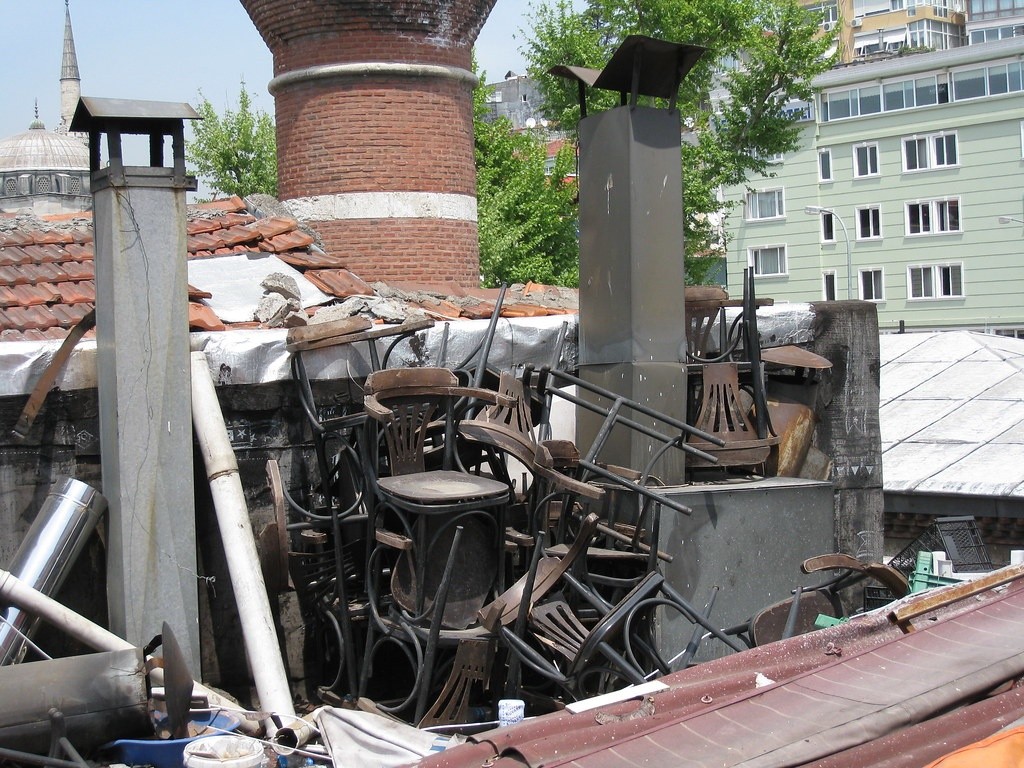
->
xmin=803 ymin=205 xmax=852 ymax=299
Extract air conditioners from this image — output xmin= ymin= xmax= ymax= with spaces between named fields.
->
xmin=851 ymin=18 xmax=862 ymax=28
xmin=823 ymin=22 xmax=834 ymax=32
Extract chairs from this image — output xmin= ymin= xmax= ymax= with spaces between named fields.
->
xmin=226 ymin=268 xmax=914 ymax=738
